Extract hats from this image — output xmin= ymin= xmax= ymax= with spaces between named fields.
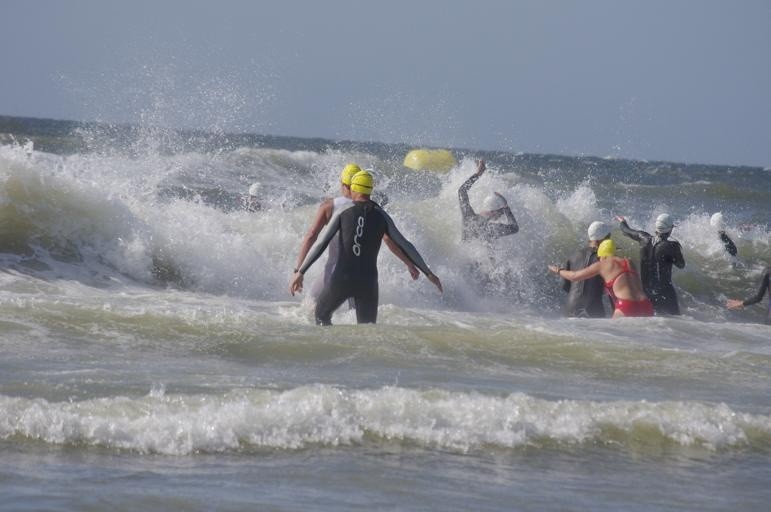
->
xmin=587 ymin=220 xmax=610 ymax=241
xmin=250 ymin=182 xmax=263 ymax=197
xmin=483 ymin=193 xmax=507 ymax=211
xmin=597 ymin=239 xmax=617 ymax=256
xmin=339 ymin=164 xmax=374 ymax=194
xmin=655 ymin=213 xmax=673 ymax=233
xmin=709 ymin=213 xmax=725 ymax=232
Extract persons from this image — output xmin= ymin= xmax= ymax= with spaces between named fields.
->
xmin=289 ymin=163 xmax=443 ymax=325
xmin=548 ymin=212 xmax=771 ymax=325
xmin=458 ymin=159 xmax=519 ymax=242
